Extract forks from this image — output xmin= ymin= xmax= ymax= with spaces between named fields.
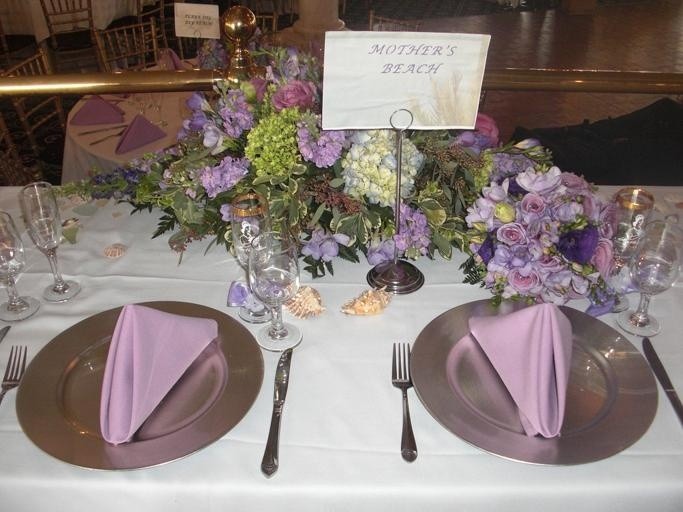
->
xmin=89 ymin=128 xmax=126 ymax=144
xmin=392 ymin=342 xmax=418 ymax=463
xmin=0 ymin=345 xmax=27 ymax=405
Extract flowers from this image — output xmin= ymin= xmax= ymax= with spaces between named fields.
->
xmin=46 ymin=47 xmax=622 ymax=309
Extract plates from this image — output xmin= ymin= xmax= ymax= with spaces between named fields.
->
xmin=15 ymin=301 xmax=265 ymax=470
xmin=409 ymin=299 xmax=659 ymax=467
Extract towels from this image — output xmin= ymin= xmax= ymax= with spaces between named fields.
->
xmin=467 ymin=300 xmax=574 ymax=441
xmin=68 ymin=96 xmax=167 ymax=155
xmin=157 ymin=49 xmax=183 ymax=71
xmin=97 ymin=302 xmax=219 ymax=443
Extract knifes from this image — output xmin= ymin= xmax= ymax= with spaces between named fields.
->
xmin=641 ymin=337 xmax=683 ymax=429
xmin=78 ymin=125 xmax=127 ymax=135
xmin=261 ymin=348 xmax=293 ymax=477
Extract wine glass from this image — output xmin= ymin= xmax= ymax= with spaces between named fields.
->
xmin=248 ymin=229 xmax=303 ymax=353
xmin=19 ymin=181 xmax=82 ymax=303
xmin=0 ymin=210 xmax=41 ymax=322
xmin=132 ymin=64 xmax=171 ymax=129
xmin=231 ymin=193 xmax=276 ymax=325
xmin=604 ymin=188 xmax=683 ymax=337
